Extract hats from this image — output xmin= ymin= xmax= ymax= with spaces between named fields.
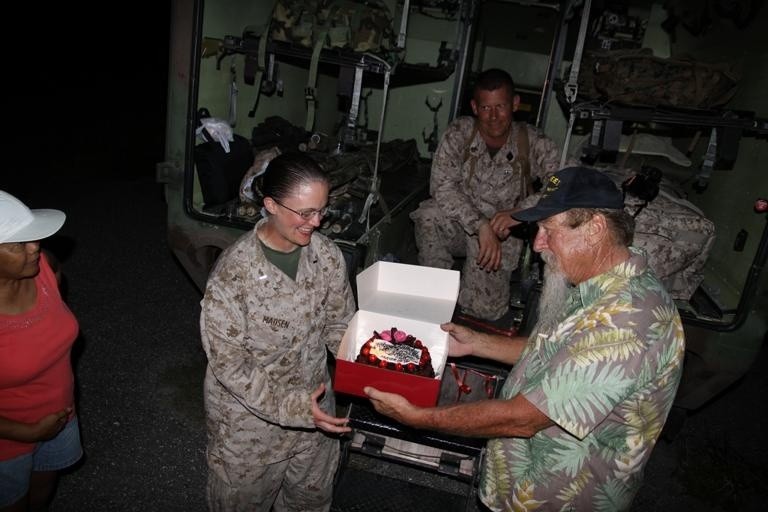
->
xmin=510 ymin=167 xmax=625 ymax=220
xmin=0 ymin=191 xmax=66 ymax=244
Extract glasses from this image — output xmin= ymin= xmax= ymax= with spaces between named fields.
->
xmin=274 ymin=199 xmax=331 ymax=220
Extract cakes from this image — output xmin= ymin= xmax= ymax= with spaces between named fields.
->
xmin=352 ymin=326 xmax=437 ymax=380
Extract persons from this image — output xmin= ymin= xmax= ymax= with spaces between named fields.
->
xmin=0 ymin=190 xmax=86 ymax=512
xmin=410 ymin=66 xmax=585 ymax=364
xmin=200 ymin=149 xmax=360 ymax=511
xmin=364 ymin=166 xmax=686 ymax=510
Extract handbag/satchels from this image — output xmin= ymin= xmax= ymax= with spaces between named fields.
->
xmin=195 ymin=134 xmax=252 ymax=214
